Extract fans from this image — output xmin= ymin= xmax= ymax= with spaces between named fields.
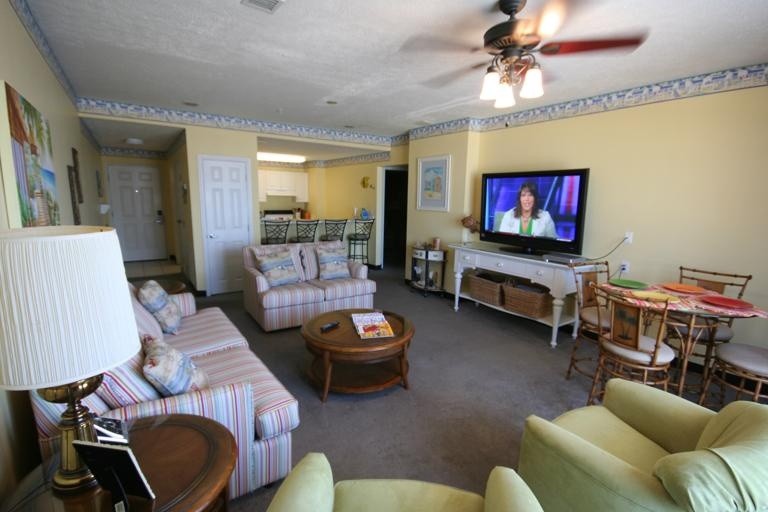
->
xmin=397 ymin=1 xmax=649 ymax=91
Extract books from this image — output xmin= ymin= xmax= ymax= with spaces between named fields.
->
xmin=352 ymin=312 xmax=395 ymax=339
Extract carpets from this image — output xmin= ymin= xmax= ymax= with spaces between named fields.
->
xmin=124 ymin=259 xmax=182 ymax=278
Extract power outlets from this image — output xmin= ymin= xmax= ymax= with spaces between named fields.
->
xmin=623 ymin=232 xmax=632 ymax=245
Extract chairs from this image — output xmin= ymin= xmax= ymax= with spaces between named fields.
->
xmin=518 ymin=378 xmax=768 ymax=512
xmin=570 ymin=259 xmax=768 ymax=418
xmin=268 ymin=451 xmax=546 ymax=511
xmin=263 ymin=217 xmax=374 ymax=270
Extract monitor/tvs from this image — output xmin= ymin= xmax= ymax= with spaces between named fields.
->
xmin=479 ymin=168 xmax=590 ymax=255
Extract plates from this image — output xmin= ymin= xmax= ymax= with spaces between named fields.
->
xmin=630 ymin=291 xmax=680 ymax=302
xmin=608 ymin=279 xmax=651 ymax=290
xmin=664 ymin=282 xmax=707 ymax=294
xmin=702 ymin=296 xmax=754 ymax=310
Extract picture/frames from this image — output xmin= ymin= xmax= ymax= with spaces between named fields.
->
xmin=416 ymin=154 xmax=450 ymax=211
xmin=70 ymin=439 xmax=155 ymax=499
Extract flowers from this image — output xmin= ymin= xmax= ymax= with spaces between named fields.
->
xmin=462 ymin=215 xmax=480 ymax=233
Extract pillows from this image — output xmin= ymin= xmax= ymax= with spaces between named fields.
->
xmin=315 ymin=247 xmax=351 ymax=281
xmin=138 ymin=280 xmax=182 ymax=336
xmin=140 ymin=334 xmax=209 ymax=398
xmin=255 ymin=249 xmax=301 ymax=288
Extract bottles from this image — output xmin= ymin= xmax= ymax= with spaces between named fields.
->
xmin=305 ymin=210 xmax=311 ymax=219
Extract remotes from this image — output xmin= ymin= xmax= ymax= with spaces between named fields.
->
xmin=321 ymin=321 xmax=340 ymax=333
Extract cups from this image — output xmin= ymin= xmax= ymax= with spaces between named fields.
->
xmin=295 ymin=212 xmax=301 ymax=219
xmin=352 ymin=208 xmax=358 ymax=219
xmin=433 ymin=237 xmax=441 ymax=251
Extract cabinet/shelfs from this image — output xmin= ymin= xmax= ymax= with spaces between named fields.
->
xmin=258 ymin=171 xmax=308 ymax=203
xmin=448 ymin=241 xmax=591 ymax=348
xmin=409 ymin=245 xmax=447 ymax=299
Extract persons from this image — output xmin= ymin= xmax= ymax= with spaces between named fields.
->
xmin=499 ymin=183 xmax=557 ymax=239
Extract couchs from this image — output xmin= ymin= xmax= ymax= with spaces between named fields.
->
xmin=28 ymin=280 xmax=303 ymax=502
xmin=243 ymin=241 xmax=376 ymax=332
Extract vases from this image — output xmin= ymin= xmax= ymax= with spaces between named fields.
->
xmin=461 ymin=227 xmax=469 ymax=243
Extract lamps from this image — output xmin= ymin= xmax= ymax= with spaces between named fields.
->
xmin=480 ymin=54 xmax=544 ymax=110
xmin=0 ymin=225 xmax=142 ymax=495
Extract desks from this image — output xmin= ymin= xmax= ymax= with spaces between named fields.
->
xmin=0 ymin=414 xmax=239 ymax=512
